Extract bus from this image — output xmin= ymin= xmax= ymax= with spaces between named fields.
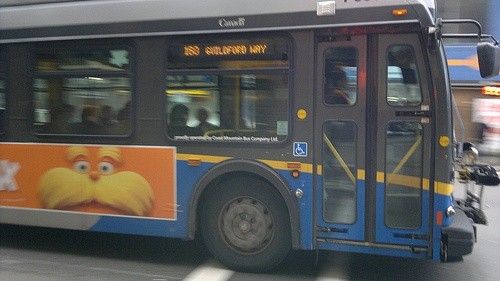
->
xmin=0 ymin=0 xmax=500 ymax=263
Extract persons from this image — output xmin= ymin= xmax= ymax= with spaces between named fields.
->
xmin=194 ymin=107 xmax=224 ymax=129
xmin=327 ymin=68 xmax=357 ymax=143
xmin=47 ymin=98 xmax=133 ymax=135
xmin=247 ymin=76 xmax=289 ymax=136
xmin=167 ymin=103 xmax=199 ymax=137
